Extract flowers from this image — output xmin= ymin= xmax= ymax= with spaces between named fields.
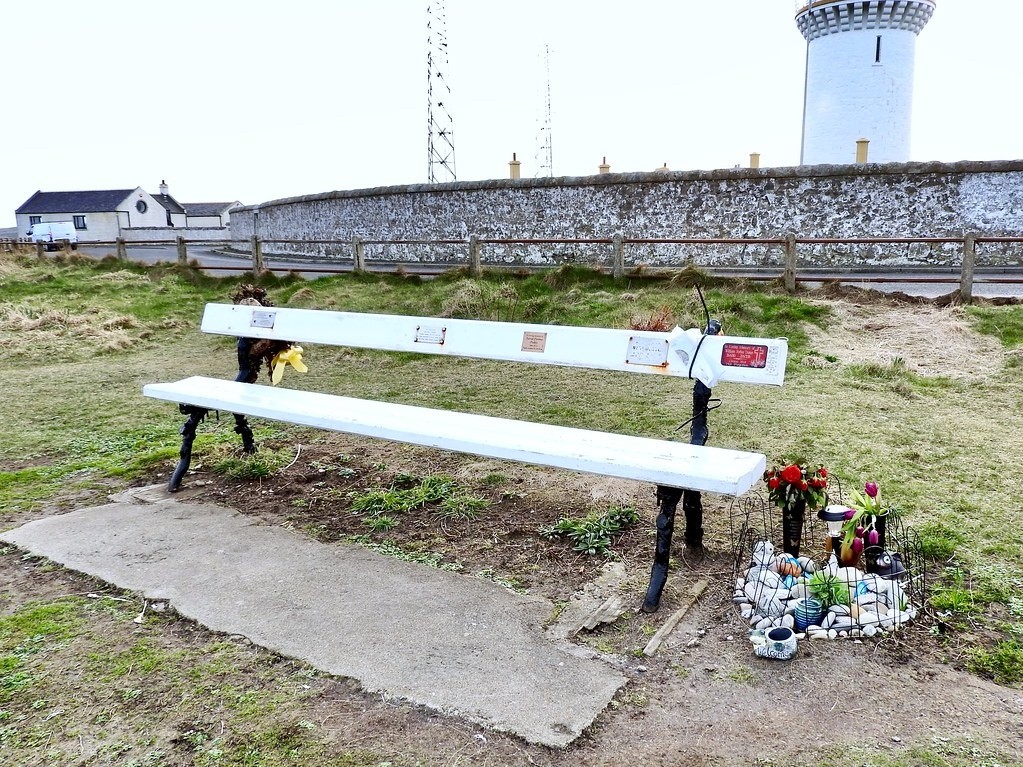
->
xmin=763 ymin=452 xmax=830 ymax=510
xmin=839 ymin=480 xmax=887 ymax=565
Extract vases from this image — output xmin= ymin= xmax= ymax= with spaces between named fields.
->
xmin=861 ymin=507 xmax=890 ymax=575
xmin=751 ymin=625 xmax=798 ymax=661
xmin=782 ymin=499 xmax=806 ymax=559
xmin=794 ymin=597 xmax=822 ymax=630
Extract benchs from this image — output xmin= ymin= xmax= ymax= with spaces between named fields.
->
xmin=142 ymin=303 xmax=789 ymax=616
